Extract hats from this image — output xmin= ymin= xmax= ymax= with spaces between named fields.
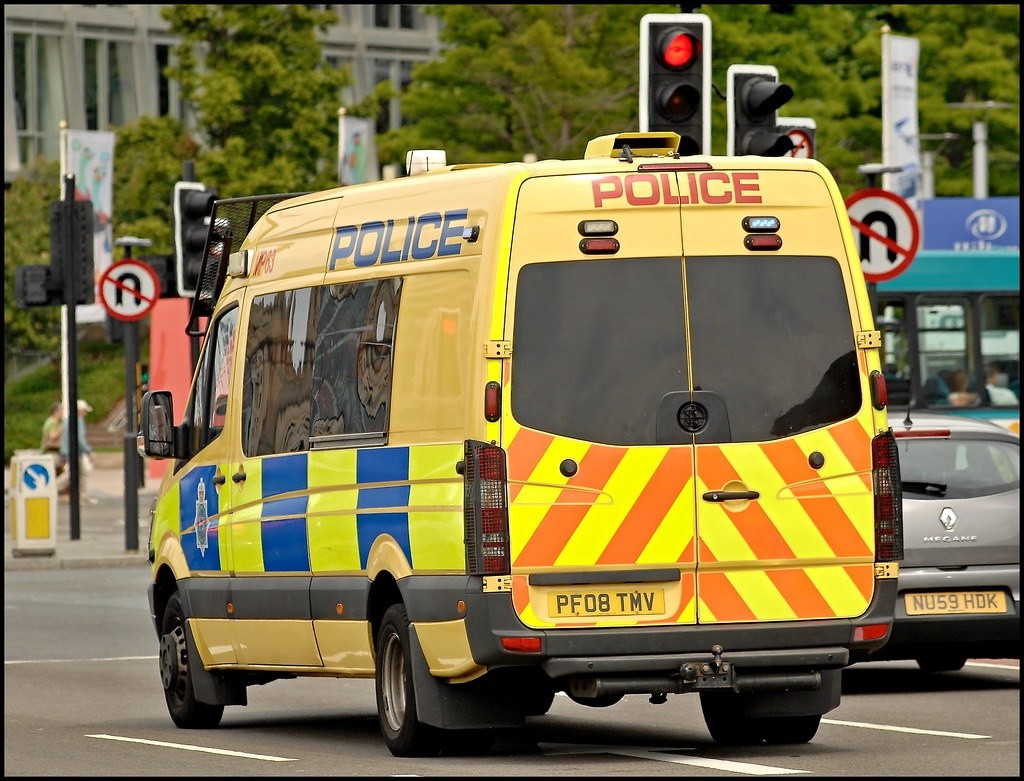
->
xmin=77 ymin=399 xmax=93 ymax=412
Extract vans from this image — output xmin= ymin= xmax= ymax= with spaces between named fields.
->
xmin=134 ymin=134 xmax=898 ymax=759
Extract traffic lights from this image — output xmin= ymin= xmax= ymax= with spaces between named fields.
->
xmin=728 ymin=66 xmax=796 ymax=157
xmin=140 ymin=364 xmax=150 ymax=392
xmin=639 ymin=15 xmax=710 ymax=153
xmin=174 ymin=182 xmax=220 ymax=298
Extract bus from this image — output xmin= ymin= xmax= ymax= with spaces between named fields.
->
xmin=865 ymin=249 xmax=1021 ymax=436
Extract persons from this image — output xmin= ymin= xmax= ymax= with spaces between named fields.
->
xmin=40 ymin=399 xmax=99 ymax=506
xmin=939 ymin=363 xmax=1019 ymax=406
xmin=349 ymin=131 xmax=368 ymax=184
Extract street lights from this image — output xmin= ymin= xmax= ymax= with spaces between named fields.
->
xmin=858 ymin=161 xmax=904 ymax=300
xmin=906 ymin=136 xmax=954 ymax=200
xmin=942 ymin=102 xmax=1010 ymax=199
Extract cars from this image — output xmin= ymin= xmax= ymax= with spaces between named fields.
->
xmin=851 ymin=413 xmax=1020 ymax=669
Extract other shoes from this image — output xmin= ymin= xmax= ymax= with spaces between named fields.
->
xmin=80 ymin=496 xmax=98 ymax=505
